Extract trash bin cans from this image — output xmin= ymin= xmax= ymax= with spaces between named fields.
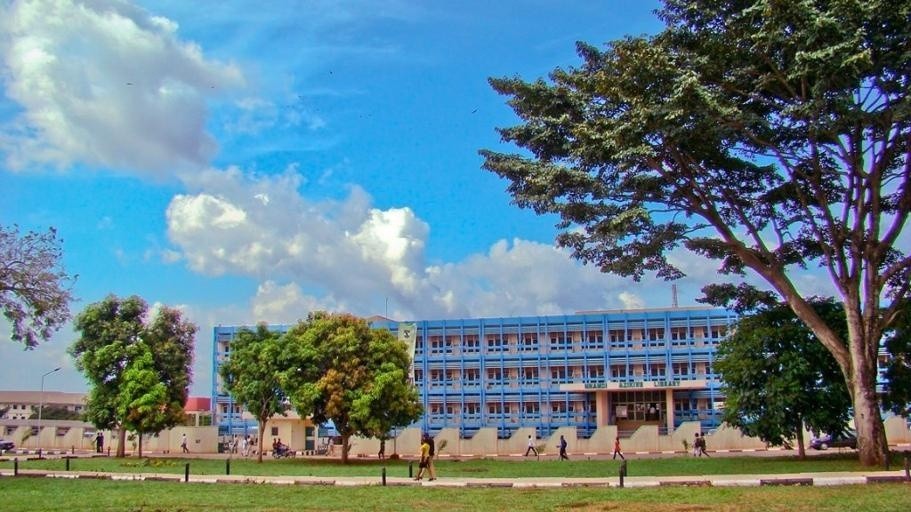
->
xmin=218 ymin=443 xmax=224 ymax=453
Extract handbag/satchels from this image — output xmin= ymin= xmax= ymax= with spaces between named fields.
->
xmin=419 ymin=460 xmax=428 ymax=469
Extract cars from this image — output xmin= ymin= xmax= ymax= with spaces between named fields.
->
xmin=811 ymin=427 xmax=860 ymax=450
xmin=1 ymin=437 xmax=14 ymax=452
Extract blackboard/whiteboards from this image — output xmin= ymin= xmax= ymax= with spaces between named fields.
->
xmin=616 ymin=406 xmax=627 ymax=417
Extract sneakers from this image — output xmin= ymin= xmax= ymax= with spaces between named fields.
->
xmin=414 ymin=476 xmax=436 ymax=482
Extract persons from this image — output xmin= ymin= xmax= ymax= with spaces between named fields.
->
xmin=556 ymin=435 xmax=568 ymax=461
xmin=276 ymin=439 xmax=281 ymax=458
xmin=526 ymin=435 xmax=537 ymax=456
xmin=325 ymin=437 xmax=335 ymax=456
xmin=272 ymin=438 xmax=277 ymax=458
xmin=420 ymin=433 xmax=437 ymax=480
xmin=181 ymin=434 xmax=188 ymax=453
xmin=693 ymin=432 xmax=709 ymax=457
xmin=243 ymin=435 xmax=251 ymax=456
xmin=100 ymin=433 xmax=104 ymax=452
xmin=614 ymin=437 xmax=624 ymax=459
xmin=415 ymin=437 xmax=433 ymax=481
xmin=231 ymin=434 xmax=238 ymax=453
xmin=92 ymin=433 xmax=101 ymax=452
xmin=903 ymin=450 xmax=911 ymax=481
xmin=378 ymin=440 xmax=384 ymax=459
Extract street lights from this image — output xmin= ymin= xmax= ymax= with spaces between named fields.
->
xmin=37 ymin=367 xmax=63 ymax=447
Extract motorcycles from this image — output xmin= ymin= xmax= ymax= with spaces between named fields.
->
xmin=270 ymin=445 xmax=298 ymax=459
